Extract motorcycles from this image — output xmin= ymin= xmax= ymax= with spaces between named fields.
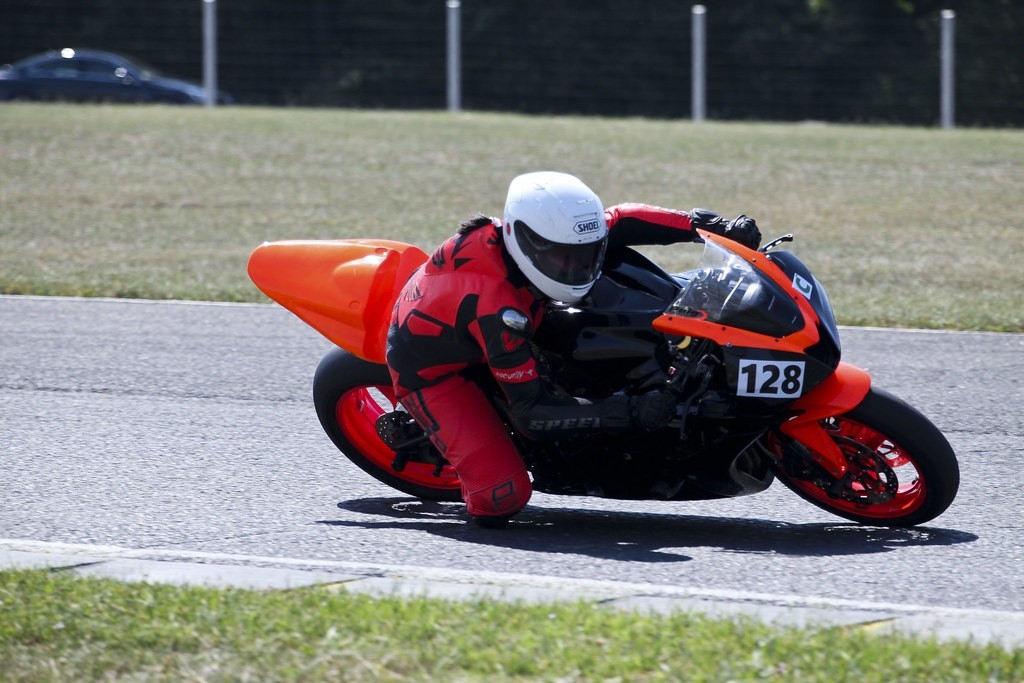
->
xmin=247 ymin=228 xmax=961 ymax=528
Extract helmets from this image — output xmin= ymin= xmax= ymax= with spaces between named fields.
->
xmin=503 ymin=172 xmax=610 ymax=305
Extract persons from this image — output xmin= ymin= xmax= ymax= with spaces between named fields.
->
xmin=386 ymin=167 xmax=760 ymax=530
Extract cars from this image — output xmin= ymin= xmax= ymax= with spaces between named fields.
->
xmin=0 ymin=49 xmax=230 ymax=106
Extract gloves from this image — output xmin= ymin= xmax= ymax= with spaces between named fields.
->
xmin=691 ymin=207 xmax=762 ymax=253
xmin=617 ymin=387 xmax=678 ymax=431
